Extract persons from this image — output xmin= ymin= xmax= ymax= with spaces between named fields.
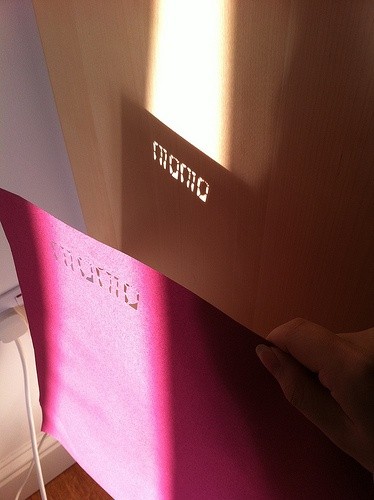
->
xmin=254 ymin=322 xmax=374 ymax=475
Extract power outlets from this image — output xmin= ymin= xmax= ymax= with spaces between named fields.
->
xmin=0 ymin=286 xmax=25 ymax=313
